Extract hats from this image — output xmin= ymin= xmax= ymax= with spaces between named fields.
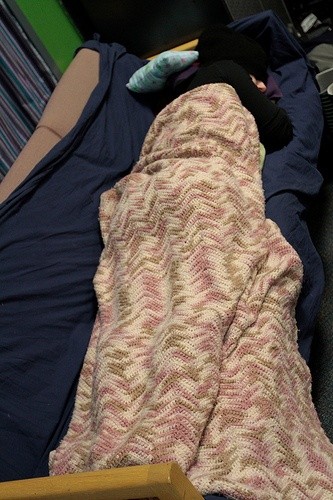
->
xmin=195 ymin=24 xmax=269 ymax=67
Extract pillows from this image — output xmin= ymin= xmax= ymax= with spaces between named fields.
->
xmin=172 ymin=62 xmax=283 ymax=99
xmin=125 ymin=50 xmax=198 ymax=93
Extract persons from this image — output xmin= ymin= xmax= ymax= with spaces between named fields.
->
xmin=184 ymin=17 xmax=295 ymax=148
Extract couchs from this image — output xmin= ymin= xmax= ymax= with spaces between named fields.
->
xmin=1 ymin=9 xmax=323 ymax=500
